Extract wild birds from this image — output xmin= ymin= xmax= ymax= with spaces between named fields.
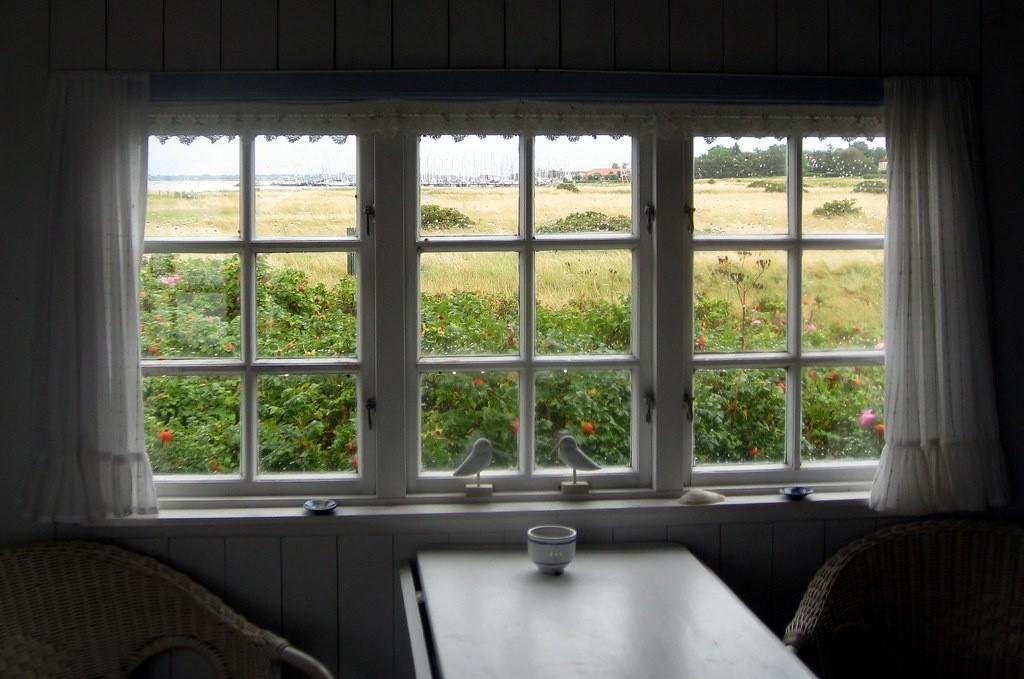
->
xmin=452 ymin=437 xmax=510 ymax=478
xmin=547 ymin=434 xmax=603 ymax=472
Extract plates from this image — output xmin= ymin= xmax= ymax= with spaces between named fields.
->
xmin=304 ymin=499 xmax=338 ymax=512
xmin=780 ymin=487 xmax=813 ymax=499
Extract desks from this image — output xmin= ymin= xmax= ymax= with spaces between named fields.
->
xmin=399 ymin=541 xmax=818 ymax=679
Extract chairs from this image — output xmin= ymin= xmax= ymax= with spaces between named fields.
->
xmin=0 ymin=541 xmax=335 ymax=679
xmin=784 ymin=517 xmax=1024 ymax=679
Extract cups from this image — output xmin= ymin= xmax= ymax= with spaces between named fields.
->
xmin=527 ymin=525 xmax=577 ymax=574
